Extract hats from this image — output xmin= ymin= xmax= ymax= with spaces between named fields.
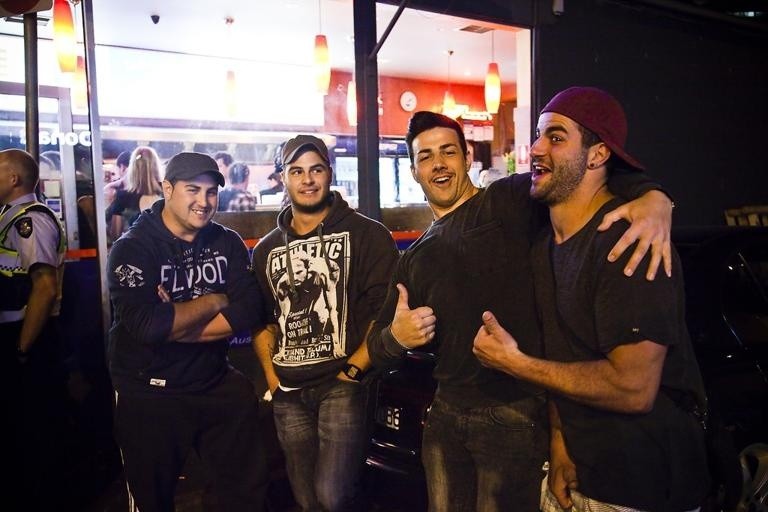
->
xmin=540 ymin=86 xmax=645 ymax=171
xmin=166 ymin=153 xmax=225 ymax=187
xmin=281 ymin=135 xmax=329 ymax=164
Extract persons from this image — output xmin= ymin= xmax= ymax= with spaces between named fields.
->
xmin=473 ymin=87 xmax=706 ymax=512
xmin=367 ymin=110 xmax=674 ymax=511
xmin=105 ymin=153 xmax=266 ymax=511
xmin=248 ymin=134 xmax=400 ymax=510
xmin=72 ymin=146 xmax=284 ymax=262
xmin=0 ymin=149 xmax=66 ymax=511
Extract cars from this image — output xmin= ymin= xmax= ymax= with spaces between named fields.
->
xmin=361 ymin=225 xmax=767 ymax=512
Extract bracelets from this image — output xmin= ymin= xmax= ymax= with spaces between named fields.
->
xmin=388 ymin=327 xmax=413 ymax=351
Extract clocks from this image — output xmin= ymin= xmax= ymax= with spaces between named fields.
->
xmin=398 ymin=88 xmax=418 ymax=112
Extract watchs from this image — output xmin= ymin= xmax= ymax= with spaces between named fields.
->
xmin=341 ymin=363 xmax=364 ymax=382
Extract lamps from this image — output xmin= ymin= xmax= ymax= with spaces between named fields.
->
xmin=440 ymin=51 xmax=454 ymax=120
xmin=483 ymin=28 xmax=503 ymax=114
xmin=52 ymin=0 xmax=78 ymax=72
xmin=313 ymin=0 xmax=330 ymax=97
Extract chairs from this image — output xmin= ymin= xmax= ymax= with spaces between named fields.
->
xmin=696 ymin=228 xmax=766 ymax=433
